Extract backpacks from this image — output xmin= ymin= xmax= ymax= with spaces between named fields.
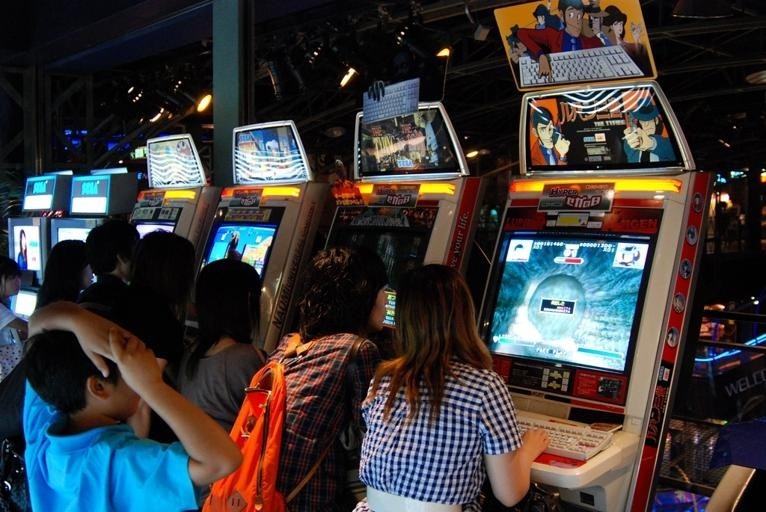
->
xmin=202 ymin=330 xmax=342 ymax=511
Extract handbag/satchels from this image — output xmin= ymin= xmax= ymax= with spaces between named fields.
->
xmin=339 ymin=337 xmax=366 ymax=501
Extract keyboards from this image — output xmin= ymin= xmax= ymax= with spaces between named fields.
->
xmin=362 ymin=77 xmax=421 ymax=127
xmin=518 ymin=44 xmax=644 ymax=89
xmin=514 ymin=417 xmax=614 ymax=461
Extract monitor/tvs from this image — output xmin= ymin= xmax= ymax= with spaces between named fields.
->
xmin=485 ymin=226 xmax=658 ymax=375
xmin=50 ymin=218 xmax=98 ymax=283
xmin=7 ymin=216 xmax=49 ymax=280
xmin=190 ymin=218 xmax=278 ymax=289
xmin=129 ymin=218 xmax=176 ymax=242
xmin=14 ymin=290 xmax=37 ymax=318
xmin=328 ymin=225 xmax=431 ymax=338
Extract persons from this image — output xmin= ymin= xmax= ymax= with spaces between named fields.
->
xmin=117 ymin=232 xmax=197 ymax=442
xmin=259 ymin=244 xmax=388 ymax=510
xmin=83 ymin=217 xmax=138 ymax=332
xmin=165 ymin=258 xmax=267 ymax=511
xmin=354 ymin=263 xmax=550 ymax=511
xmin=0 ymin=257 xmax=27 ymax=383
xmin=38 ymin=239 xmax=90 ymax=303
xmin=22 ymin=303 xmax=241 ymax=510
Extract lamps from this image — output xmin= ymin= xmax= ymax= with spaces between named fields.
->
xmin=118 ymin=0 xmax=453 ymax=123
